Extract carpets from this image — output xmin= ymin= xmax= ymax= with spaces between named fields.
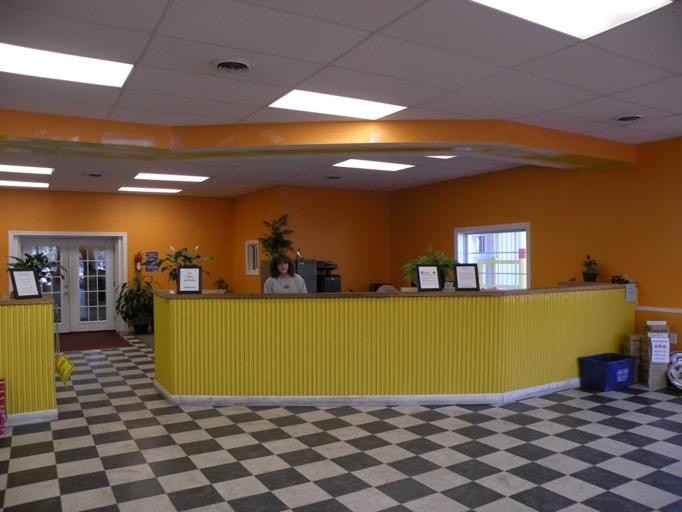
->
xmin=54 ymin=331 xmax=134 ymax=355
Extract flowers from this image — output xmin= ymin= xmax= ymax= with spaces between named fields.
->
xmin=156 ymin=244 xmax=215 ymax=281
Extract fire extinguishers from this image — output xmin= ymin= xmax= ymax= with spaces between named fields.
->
xmin=134 ymin=250 xmax=144 ymax=271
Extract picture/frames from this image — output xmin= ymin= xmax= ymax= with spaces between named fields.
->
xmin=8 ymin=268 xmax=42 ymax=299
xmin=416 ymin=263 xmax=480 ymax=291
xmin=177 ymin=266 xmax=202 ymax=295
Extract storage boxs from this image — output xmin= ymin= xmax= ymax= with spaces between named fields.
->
xmin=574 ymin=326 xmax=671 ymax=393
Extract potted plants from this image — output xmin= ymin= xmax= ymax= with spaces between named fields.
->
xmin=401 ymin=250 xmax=458 ymax=291
xmin=113 ymin=275 xmax=155 ymax=336
xmin=257 ymin=213 xmax=296 ymax=258
xmin=579 ymin=254 xmax=602 ymax=282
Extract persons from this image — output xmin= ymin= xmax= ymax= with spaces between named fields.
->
xmin=263 ymin=253 xmax=309 ymax=294
